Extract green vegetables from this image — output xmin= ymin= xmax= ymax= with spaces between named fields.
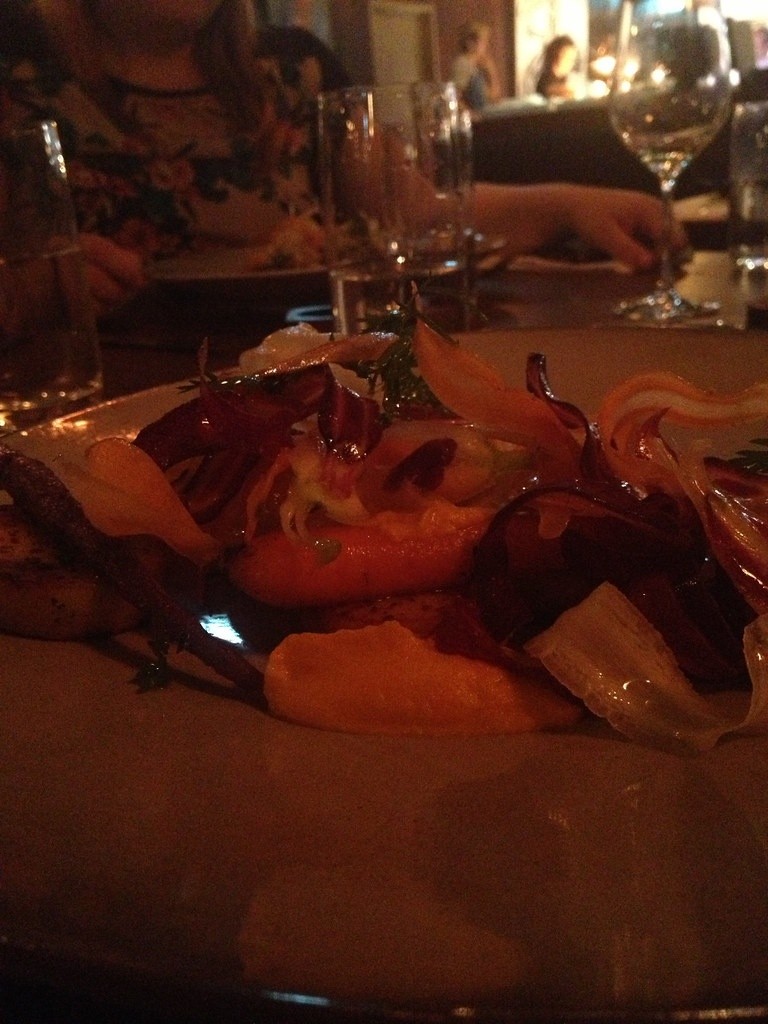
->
xmin=172 ymin=273 xmax=487 ymax=417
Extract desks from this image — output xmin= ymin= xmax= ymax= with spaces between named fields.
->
xmin=472 ymin=65 xmax=768 ymax=205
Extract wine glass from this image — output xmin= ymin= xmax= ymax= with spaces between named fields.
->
xmin=609 ymin=0 xmax=731 ymax=322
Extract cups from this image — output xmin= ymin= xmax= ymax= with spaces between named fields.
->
xmin=731 ymin=101 xmax=768 ymax=309
xmin=0 ymin=121 xmax=113 ymax=410
xmin=318 ymin=81 xmax=475 ymax=334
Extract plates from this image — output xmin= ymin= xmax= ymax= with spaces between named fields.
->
xmin=1 ymin=330 xmax=768 ymax=1024
xmin=145 ymin=247 xmax=327 ymax=279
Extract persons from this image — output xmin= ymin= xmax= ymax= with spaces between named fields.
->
xmin=1 ymin=0 xmax=689 ymax=395
xmin=536 ymin=36 xmax=582 ymax=98
xmin=445 ymin=18 xmax=503 ymax=124
